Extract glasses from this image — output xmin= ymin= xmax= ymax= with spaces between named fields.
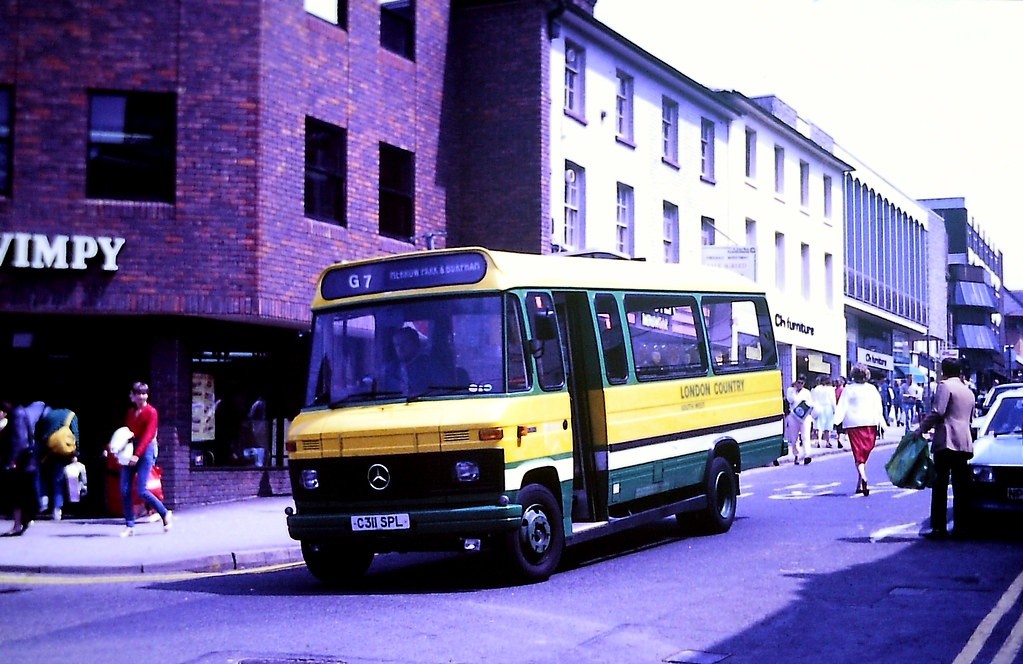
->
xmin=797 ymin=381 xmax=805 ymax=385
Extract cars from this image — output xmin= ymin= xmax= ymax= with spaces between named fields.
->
xmin=967 ymin=380 xmax=1023 ymax=523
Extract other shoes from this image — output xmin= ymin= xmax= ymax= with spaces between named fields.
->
xmin=807 ymin=414 xmax=939 ymax=451
xmin=804 ymin=457 xmax=811 ymax=465
xmin=0 ymin=515 xmax=34 ymax=537
xmin=861 ymin=479 xmax=869 ymax=496
xmin=855 ymin=487 xmax=864 ymax=494
xmin=51 ymin=509 xmax=62 ymax=520
xmin=950 ymin=529 xmax=965 ymax=539
xmin=793 ymin=456 xmax=800 ymax=465
xmin=39 ymin=495 xmax=48 ymax=513
xmin=918 ymin=531 xmax=950 ymax=540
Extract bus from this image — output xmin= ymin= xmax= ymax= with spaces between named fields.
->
xmin=282 ymin=245 xmax=791 ymax=584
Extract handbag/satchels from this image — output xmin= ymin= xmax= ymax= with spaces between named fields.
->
xmin=64 ymin=457 xmax=88 ymax=502
xmin=48 ymin=425 xmax=76 ymax=456
xmin=884 ymin=426 xmax=937 ymax=490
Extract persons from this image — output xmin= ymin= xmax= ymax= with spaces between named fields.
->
xmin=834 ymin=365 xmax=887 ymax=497
xmin=234 ymin=396 xmax=268 ymax=465
xmin=369 ymin=325 xmax=438 ymax=400
xmin=914 ymin=357 xmax=973 ymax=540
xmin=808 ymin=371 xmax=987 ymax=449
xmin=23 ymin=396 xmax=79 ymax=520
xmin=772 ymin=372 xmax=813 ymax=467
xmin=119 ymin=382 xmax=173 ymax=534
xmin=0 ymin=390 xmax=39 ymax=536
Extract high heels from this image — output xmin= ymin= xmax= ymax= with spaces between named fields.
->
xmin=120 ymin=526 xmax=135 ymax=537
xmin=163 ymin=511 xmax=175 ymax=531
